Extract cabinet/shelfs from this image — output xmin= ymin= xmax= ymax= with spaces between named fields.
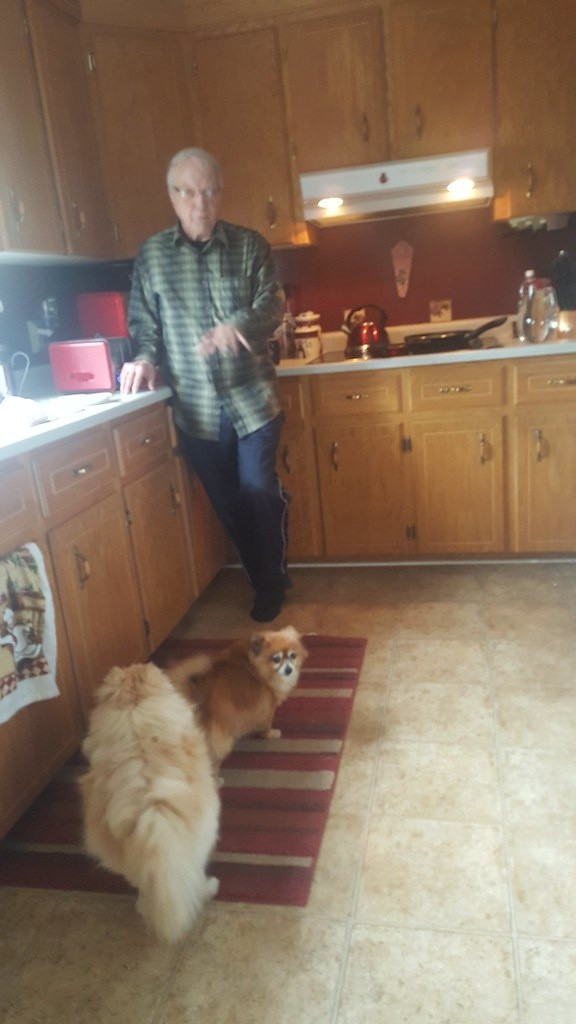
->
xmin=0 ymin=0 xmax=576 ymax=268
xmin=0 ymin=351 xmax=576 ymax=843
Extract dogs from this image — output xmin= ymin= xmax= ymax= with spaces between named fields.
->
xmin=77 ymin=625 xmax=309 ymax=952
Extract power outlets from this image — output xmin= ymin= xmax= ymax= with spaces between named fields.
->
xmin=428 ymin=299 xmax=452 ymax=323
xmin=343 ymin=309 xmax=366 ymax=324
xmin=42 ymin=296 xmax=60 ymax=331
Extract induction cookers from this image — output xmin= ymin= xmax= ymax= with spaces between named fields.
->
xmin=305 ymin=335 xmax=504 ymax=365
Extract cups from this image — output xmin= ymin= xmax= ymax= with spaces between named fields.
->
xmin=269 ymin=340 xmax=280 ymax=365
xmin=293 ymin=311 xmax=324 ymax=360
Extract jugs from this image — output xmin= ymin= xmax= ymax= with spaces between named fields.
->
xmin=524 ymin=278 xmax=557 ymax=345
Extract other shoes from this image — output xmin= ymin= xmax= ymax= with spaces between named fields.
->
xmin=251 ymin=583 xmax=283 ymax=621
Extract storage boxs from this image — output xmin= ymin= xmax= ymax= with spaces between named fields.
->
xmin=47 ymin=336 xmax=133 ymax=392
xmin=77 ymin=290 xmax=131 ymax=337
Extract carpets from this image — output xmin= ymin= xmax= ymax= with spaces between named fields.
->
xmin=0 ymin=636 xmax=370 ymax=908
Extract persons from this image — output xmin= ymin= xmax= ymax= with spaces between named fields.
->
xmin=118 ymin=146 xmax=292 ymax=623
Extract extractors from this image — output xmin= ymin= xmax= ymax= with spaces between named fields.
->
xmin=298 ymin=147 xmax=494 ymax=228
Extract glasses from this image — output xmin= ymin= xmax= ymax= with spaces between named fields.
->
xmin=172 ymin=185 xmax=221 ymax=201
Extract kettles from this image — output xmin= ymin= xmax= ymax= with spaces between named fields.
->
xmin=340 ymin=304 xmax=392 ymax=359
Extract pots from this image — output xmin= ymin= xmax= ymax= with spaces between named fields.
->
xmin=405 ymin=317 xmax=508 ymax=353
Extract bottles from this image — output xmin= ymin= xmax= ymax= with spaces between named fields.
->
xmin=517 ymin=270 xmax=537 ymax=342
xmin=282 ymin=316 xmax=293 ymax=359
